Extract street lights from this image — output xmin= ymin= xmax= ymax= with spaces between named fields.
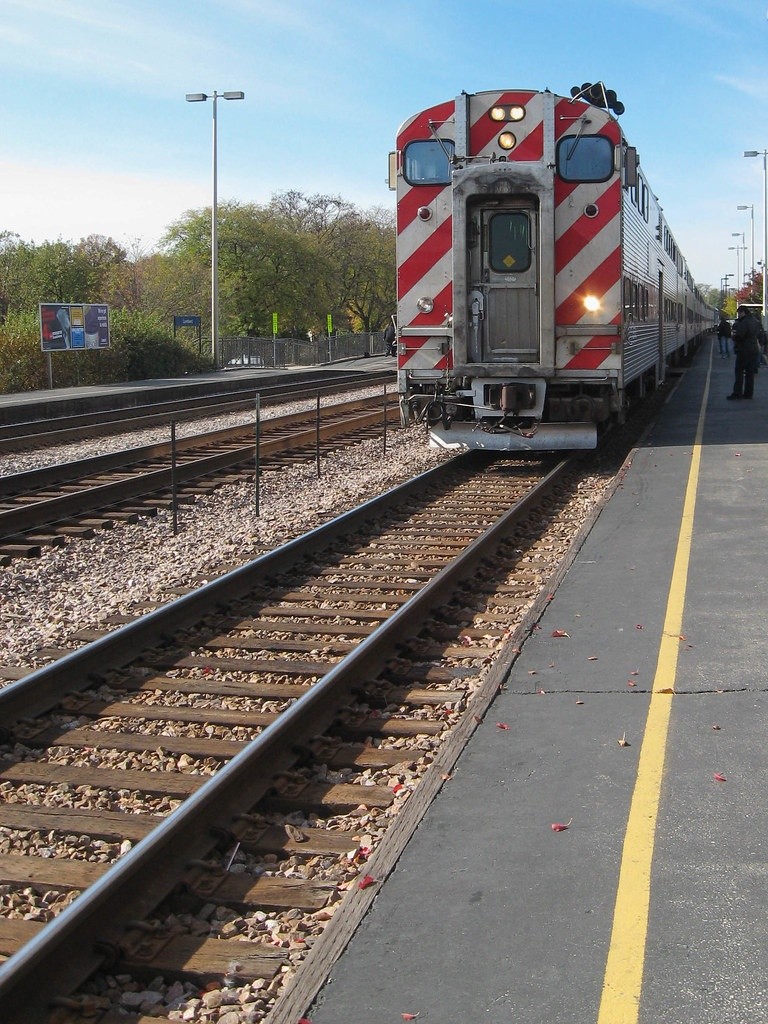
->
xmin=185 ymin=90 xmax=245 ymax=370
xmin=728 ymin=245 xmax=740 ymax=291
xmin=721 ymin=274 xmax=735 ymax=309
xmin=737 ymin=204 xmax=754 ymax=304
xmin=732 ymin=232 xmax=745 ymax=289
xmin=742 ymin=149 xmax=768 ymax=336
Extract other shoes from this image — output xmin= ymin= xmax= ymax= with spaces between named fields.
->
xmin=743 ymin=394 xmax=752 ymax=399
xmin=392 ymin=354 xmax=395 ymax=357
xmin=727 ymin=393 xmax=742 ymax=400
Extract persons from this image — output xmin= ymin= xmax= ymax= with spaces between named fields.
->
xmin=382 ymin=320 xmax=397 ymax=357
xmin=727 ymin=305 xmax=768 ymax=400
xmin=717 ymin=323 xmax=727 ymax=354
xmin=719 ymin=318 xmax=730 ymax=360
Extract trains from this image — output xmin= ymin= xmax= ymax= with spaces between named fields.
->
xmin=388 ymin=80 xmax=720 ymax=452
